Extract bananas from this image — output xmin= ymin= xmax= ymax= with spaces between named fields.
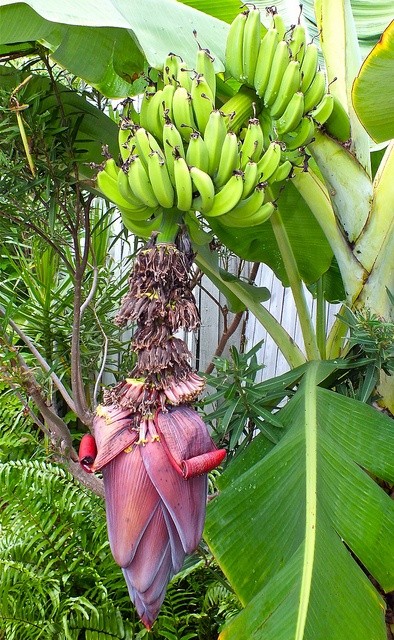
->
xmin=226 ymin=0 xmax=334 ymax=152
xmin=92 ymin=48 xmax=291 ymax=239
